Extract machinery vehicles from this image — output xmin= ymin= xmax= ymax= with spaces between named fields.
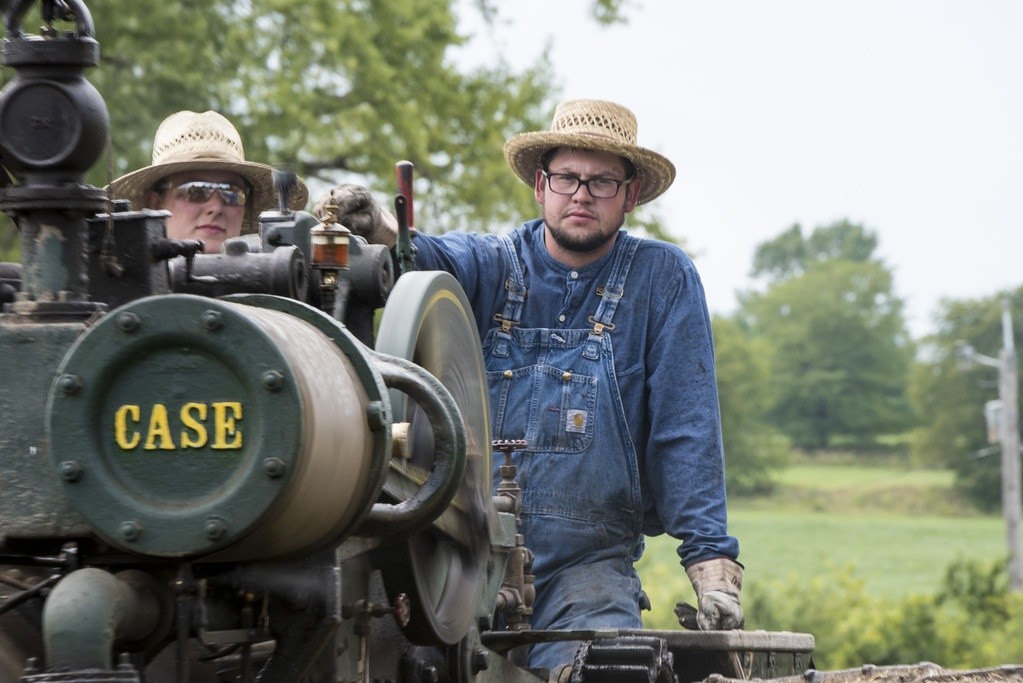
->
xmin=0 ymin=1 xmax=814 ymax=683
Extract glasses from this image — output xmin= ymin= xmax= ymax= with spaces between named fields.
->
xmin=154 ymin=178 xmax=252 ymax=207
xmin=541 ymin=161 xmax=636 ymax=199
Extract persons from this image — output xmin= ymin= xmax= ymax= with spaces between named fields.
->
xmin=312 ymin=98 xmax=744 ymax=674
xmin=103 ymin=111 xmax=309 ymax=253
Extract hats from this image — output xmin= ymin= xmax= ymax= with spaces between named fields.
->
xmin=502 ymin=99 xmax=677 ymax=206
xmin=102 ymin=109 xmax=310 ymax=236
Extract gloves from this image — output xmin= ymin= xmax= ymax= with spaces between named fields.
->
xmin=674 ymin=556 xmax=745 ymax=630
xmin=312 ymin=179 xmax=417 ymax=251
